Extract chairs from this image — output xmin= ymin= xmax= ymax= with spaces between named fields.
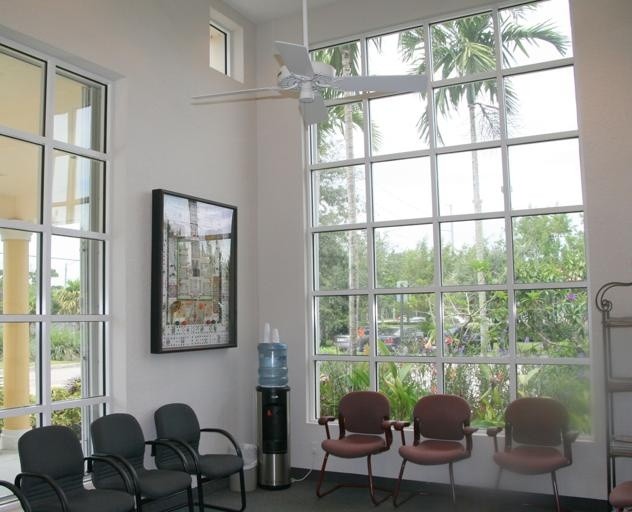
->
xmin=485 ymin=396 xmax=581 ymax=511
xmin=393 ymin=393 xmax=480 ymax=512
xmin=1 ymin=479 xmax=32 ymax=510
xmin=86 ymin=411 xmax=196 ymax=511
xmin=314 ymin=388 xmax=398 ymax=505
xmin=150 ymin=401 xmax=247 ymax=510
xmin=16 ymin=422 xmax=140 ymax=511
xmin=607 ymin=481 xmax=632 ymax=511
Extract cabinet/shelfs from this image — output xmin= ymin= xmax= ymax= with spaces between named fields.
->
xmin=595 ymin=281 xmax=632 ymax=511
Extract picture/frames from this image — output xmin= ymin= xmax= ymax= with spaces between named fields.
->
xmin=149 ymin=188 xmax=239 ymax=356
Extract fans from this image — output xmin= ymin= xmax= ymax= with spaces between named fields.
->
xmin=187 ymin=1 xmax=428 ymax=127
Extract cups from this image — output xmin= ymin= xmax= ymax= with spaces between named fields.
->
xmin=264 ymin=322 xmax=280 ymax=343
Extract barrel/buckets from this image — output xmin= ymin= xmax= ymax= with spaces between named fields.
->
xmin=228 ymin=443 xmax=259 ymax=493
xmin=257 ymin=342 xmax=290 ymax=386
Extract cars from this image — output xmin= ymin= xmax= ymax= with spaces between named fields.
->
xmin=328 ymin=314 xmax=488 ymax=355
